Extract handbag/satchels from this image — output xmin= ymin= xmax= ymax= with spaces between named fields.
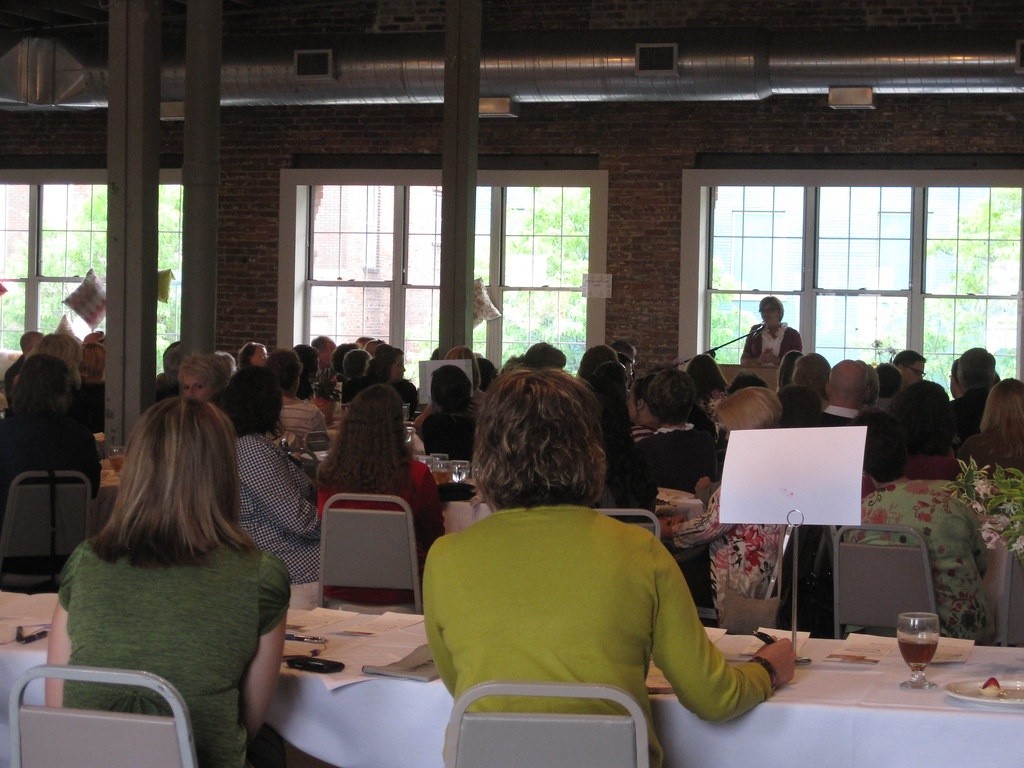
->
xmin=782 ymin=576 xmax=847 ymax=640
xmin=720 ymin=590 xmax=779 ymax=635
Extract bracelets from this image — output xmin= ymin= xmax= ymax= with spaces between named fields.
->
xmin=749 ymin=656 xmax=777 ymax=690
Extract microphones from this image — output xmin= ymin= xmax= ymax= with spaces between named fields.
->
xmin=753 ymin=322 xmax=768 ymax=337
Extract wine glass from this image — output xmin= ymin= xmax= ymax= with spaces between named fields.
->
xmin=897 ymin=612 xmax=939 ymax=690
xmin=109 ymin=446 xmax=125 ymax=475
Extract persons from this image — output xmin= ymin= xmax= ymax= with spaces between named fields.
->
xmin=225 ymin=367 xmax=322 ymax=584
xmin=578 ymin=339 xmax=1024 ymax=522
xmin=318 ymin=384 xmax=445 ymax=604
xmin=422 ymin=365 xmax=796 ymax=768
xmin=659 ymin=387 xmax=787 ymax=610
xmin=740 ymin=297 xmax=803 ymax=366
xmin=0 ymin=330 xmax=106 ymax=521
xmin=155 ymin=336 xmax=567 ymax=461
xmin=45 ymin=396 xmax=292 ymax=768
xmin=843 ymin=406 xmax=996 ymax=645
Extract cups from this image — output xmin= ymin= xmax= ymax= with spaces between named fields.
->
xmin=417 ymin=453 xmax=470 ymax=483
xmin=407 ymin=427 xmax=416 ymax=442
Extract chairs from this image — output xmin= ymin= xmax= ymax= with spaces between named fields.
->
xmin=442 ymin=679 xmax=650 ymax=768
xmin=284 ymin=431 xmax=332 ymax=470
xmin=0 ymin=468 xmax=91 ymax=592
xmin=8 ymin=664 xmax=198 ymax=768
xmin=317 ymin=495 xmax=423 ymax=615
xmin=695 ymin=524 xmax=938 ymax=640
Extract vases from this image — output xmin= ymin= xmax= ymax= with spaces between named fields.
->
xmin=311 ymin=396 xmax=337 ymax=426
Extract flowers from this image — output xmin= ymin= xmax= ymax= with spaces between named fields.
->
xmin=937 ymin=456 xmax=1024 ymax=570
xmin=308 ymin=365 xmax=345 ymax=404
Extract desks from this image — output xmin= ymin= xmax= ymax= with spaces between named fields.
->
xmin=0 ymin=637 xmax=1024 ymax=768
xmin=89 ymin=479 xmax=118 ymax=535
xmin=442 ymin=489 xmax=705 ymax=536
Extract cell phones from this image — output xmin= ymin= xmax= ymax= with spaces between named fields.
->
xmin=286 ymin=656 xmax=346 ymax=674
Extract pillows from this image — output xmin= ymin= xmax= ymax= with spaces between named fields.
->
xmin=60 ymin=268 xmax=106 ymax=332
xmin=473 ymin=277 xmax=502 ymax=328
xmin=55 ymin=314 xmax=83 ymax=346
xmin=157 ymin=268 xmax=175 ymax=304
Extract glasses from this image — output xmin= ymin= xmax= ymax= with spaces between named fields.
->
xmin=900 ymin=361 xmax=925 ymax=376
xmin=762 ymin=307 xmax=779 ymax=314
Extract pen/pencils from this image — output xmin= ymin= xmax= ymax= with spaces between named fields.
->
xmin=753 ymin=631 xmax=774 ymax=644
xmin=22 ymin=631 xmax=47 ymax=644
xmin=285 ymin=634 xmax=326 ymax=644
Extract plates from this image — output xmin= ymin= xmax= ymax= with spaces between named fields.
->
xmin=943 ymin=678 xmax=1024 ymax=704
